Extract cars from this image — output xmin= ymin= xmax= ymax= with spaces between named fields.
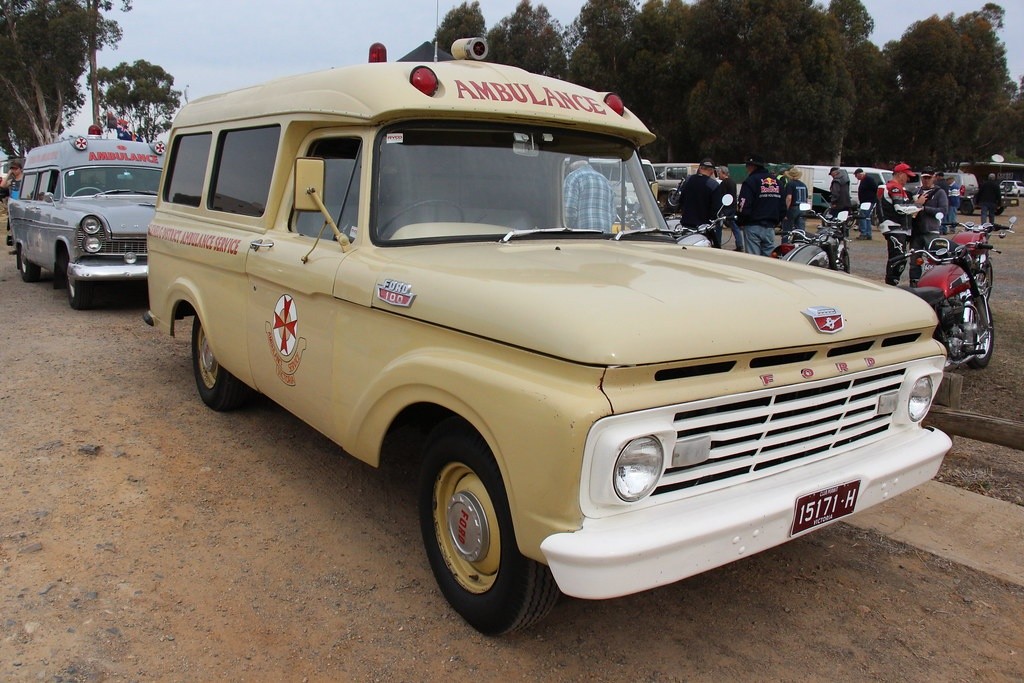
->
xmin=810 ymin=164 xmax=979 ymax=214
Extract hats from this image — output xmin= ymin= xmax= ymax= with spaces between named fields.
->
xmin=920 ymin=170 xmax=936 ymax=177
xmin=936 ymin=172 xmax=945 ymax=177
xmin=745 ymin=153 xmax=766 ymax=166
xmin=784 ymin=168 xmax=803 ymax=180
xmin=10 ymin=162 xmax=21 ymax=169
xmin=946 ymin=175 xmax=955 ymax=179
xmin=700 ymin=157 xmax=716 ymax=170
xmin=568 ymin=156 xmax=590 ymax=163
xmin=828 ymin=167 xmax=839 ymax=175
xmin=853 ymin=168 xmax=863 ymax=174
xmin=988 ymin=173 xmax=996 ymax=181
xmin=893 ymin=163 xmax=916 ymax=177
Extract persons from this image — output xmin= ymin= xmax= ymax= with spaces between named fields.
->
xmin=561 ymin=157 xmax=617 ymax=234
xmin=678 ymin=153 xmax=1005 ymax=258
xmin=0 ymin=161 xmax=23 ymax=200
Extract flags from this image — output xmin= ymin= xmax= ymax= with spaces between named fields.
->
xmin=116 ymin=123 xmax=143 ymax=141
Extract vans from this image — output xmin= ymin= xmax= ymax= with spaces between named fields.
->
xmin=587 ymin=157 xmax=659 ymax=221
xmin=651 ymin=162 xmax=716 ymax=209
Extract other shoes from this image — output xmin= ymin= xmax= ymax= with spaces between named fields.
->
xmin=856 ymin=235 xmax=872 ymax=240
xmin=735 ymin=244 xmax=743 ymax=251
xmin=8 ymin=249 xmax=17 ymax=255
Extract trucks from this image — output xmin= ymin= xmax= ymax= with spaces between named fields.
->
xmin=725 ymin=163 xmax=815 ymax=226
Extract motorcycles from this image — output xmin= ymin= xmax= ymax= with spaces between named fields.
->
xmin=769 ymin=202 xmax=871 ymax=275
xmin=665 ymin=193 xmax=738 ymax=249
xmin=874 ymin=212 xmax=1017 ymax=369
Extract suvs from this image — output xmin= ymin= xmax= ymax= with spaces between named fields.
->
xmin=7 ymin=125 xmax=168 ymax=310
xmin=145 ymin=37 xmax=951 ymax=642
xmin=999 ymin=180 xmax=1023 ymax=197
